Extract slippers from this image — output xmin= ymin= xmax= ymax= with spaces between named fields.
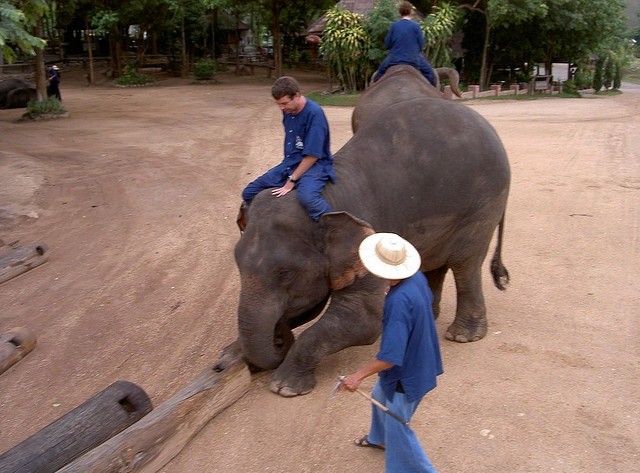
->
xmin=354 ymin=435 xmax=385 ymax=450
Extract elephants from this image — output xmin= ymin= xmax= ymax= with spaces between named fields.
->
xmin=351 ymin=63 xmax=465 ymax=135
xmin=233 ymin=96 xmax=511 ymax=398
xmin=0 ymin=77 xmax=37 ymax=109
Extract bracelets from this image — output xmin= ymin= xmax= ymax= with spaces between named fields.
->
xmin=287 ymin=175 xmax=297 ymax=184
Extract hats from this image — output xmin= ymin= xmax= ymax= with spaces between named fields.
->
xmin=359 ymin=233 xmax=422 ymax=279
xmin=52 ymin=65 xmax=59 ymax=70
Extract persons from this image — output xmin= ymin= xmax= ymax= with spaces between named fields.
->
xmin=242 ymin=76 xmax=335 ymax=222
xmin=374 ymin=2 xmax=438 ymax=88
xmin=45 ymin=64 xmax=59 ymax=100
xmin=52 ymin=65 xmax=62 ymax=100
xmin=335 ymin=231 xmax=446 ymax=471
